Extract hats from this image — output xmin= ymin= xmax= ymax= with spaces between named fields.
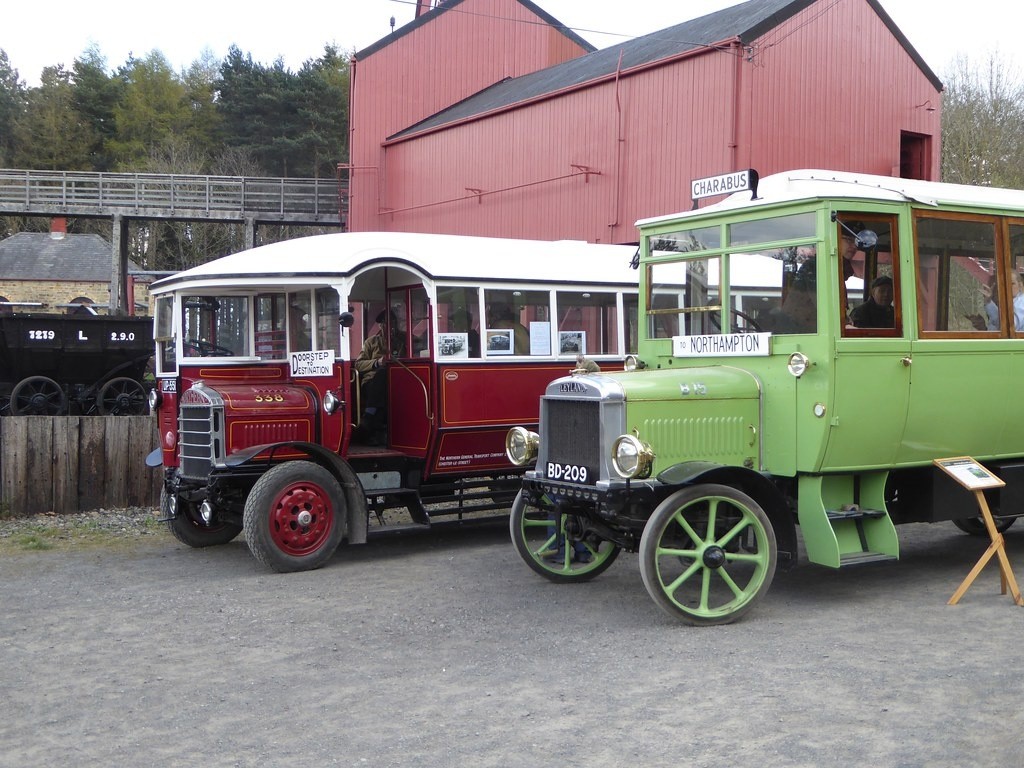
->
xmin=448 ymin=310 xmax=473 ymax=322
xmin=376 ymin=309 xmax=396 ymax=322
xmin=842 ymin=221 xmax=865 ymax=237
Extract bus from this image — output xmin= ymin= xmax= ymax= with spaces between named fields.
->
xmin=143 ymin=231 xmax=864 ymax=572
xmin=504 ymin=170 xmax=1023 ymax=627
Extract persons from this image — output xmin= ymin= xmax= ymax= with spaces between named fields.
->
xmin=771 ymin=219 xmax=866 ymax=335
xmin=274 ymin=306 xmax=311 ymax=358
xmin=849 ymin=274 xmax=895 ymax=328
xmin=487 ymin=301 xmax=530 ymax=356
xmin=447 ymin=310 xmax=480 ymax=358
xmin=354 ymin=309 xmax=407 ymax=435
xmin=964 ymin=268 xmax=1024 ymax=332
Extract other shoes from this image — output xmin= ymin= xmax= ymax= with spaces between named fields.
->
xmin=360 ymin=413 xmax=374 ymax=430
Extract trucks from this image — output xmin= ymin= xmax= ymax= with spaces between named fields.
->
xmin=0 ymin=311 xmax=155 ymax=415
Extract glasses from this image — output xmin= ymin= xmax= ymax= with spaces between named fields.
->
xmin=842 ymin=237 xmax=855 ymax=245
